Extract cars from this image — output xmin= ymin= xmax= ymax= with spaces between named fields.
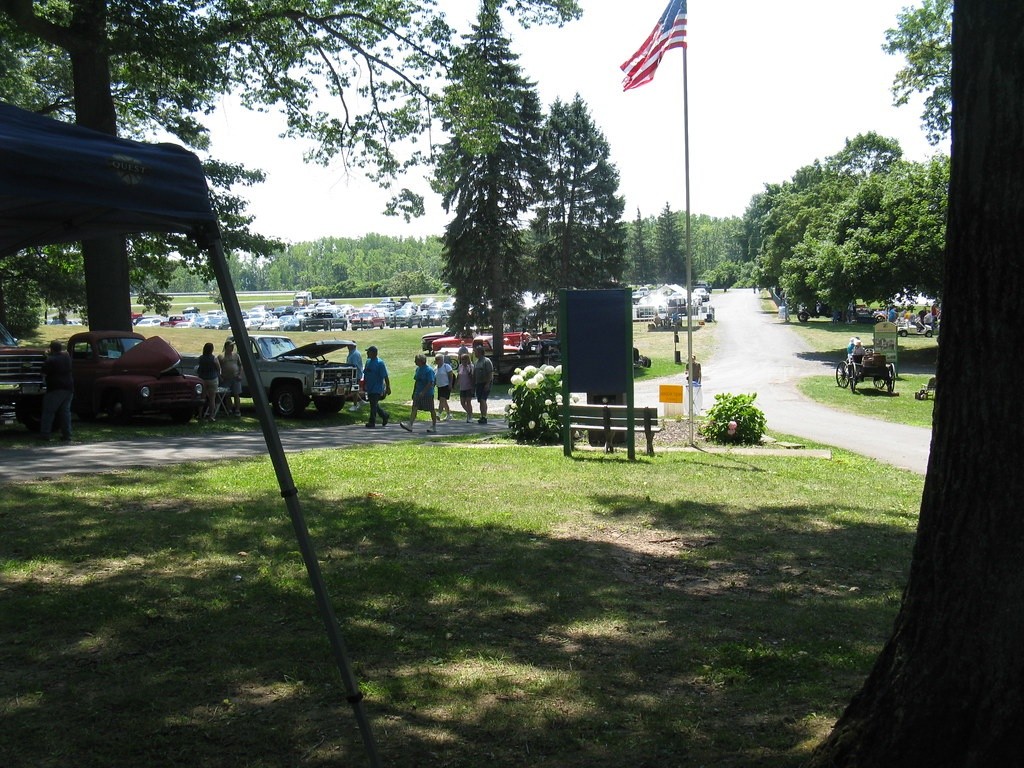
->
xmin=44 ymin=284 xmax=560 ymax=386
xmin=0 ymin=319 xmax=59 ymax=435
xmin=631 ymin=279 xmax=711 ymax=318
xmin=797 ymin=297 xmax=887 ymax=324
xmin=177 ymin=334 xmax=360 ymax=419
xmin=67 ymin=330 xmax=207 ymax=423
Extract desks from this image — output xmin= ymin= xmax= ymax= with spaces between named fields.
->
xmin=203 ymin=390 xmax=231 ymax=418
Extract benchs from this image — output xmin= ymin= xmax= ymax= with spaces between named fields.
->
xmin=556 ymin=403 xmax=662 ymax=456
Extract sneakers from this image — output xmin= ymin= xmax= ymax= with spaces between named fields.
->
xmin=234 ymin=409 xmax=242 ymax=417
xmin=348 ymin=406 xmax=357 ymax=412
xmin=400 ymin=421 xmax=413 ymax=432
xmin=356 ymin=401 xmax=367 ymax=410
xmin=436 ymin=415 xmax=441 ymax=422
xmin=443 ymin=415 xmax=451 ymax=421
xmin=467 ymin=416 xmax=473 ymax=423
xmin=426 ymin=425 xmax=437 ymax=433
xmin=365 ymin=422 xmax=375 ymax=427
xmin=477 ymin=416 xmax=487 ymax=424
xmin=382 ymin=414 xmax=390 ymax=426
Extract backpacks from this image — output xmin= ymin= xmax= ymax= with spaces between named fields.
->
xmin=444 ymin=362 xmax=456 ymax=388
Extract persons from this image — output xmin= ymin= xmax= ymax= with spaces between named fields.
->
xmin=32 ymin=340 xmax=75 ymax=445
xmin=508 ymin=316 xmax=560 ymax=367
xmin=217 ymin=339 xmax=243 ymax=418
xmin=399 ymin=353 xmax=439 ymax=433
xmin=822 ymin=292 xmax=942 ymax=386
xmin=345 ymin=339 xmax=367 ymax=412
xmin=470 ymin=344 xmax=495 ymax=425
xmin=197 ymin=342 xmax=217 ymax=424
xmin=362 ymin=346 xmax=391 ymax=428
xmin=684 ymin=355 xmax=705 ymax=411
xmin=430 ymin=353 xmax=456 ymax=420
xmin=458 ymin=340 xmax=472 ymax=366
xmin=648 ymin=306 xmax=691 ymax=332
xmin=452 ymin=354 xmax=477 ymax=424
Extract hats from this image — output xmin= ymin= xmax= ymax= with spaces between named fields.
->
xmin=365 ymin=346 xmax=377 ymax=352
xmin=224 ymin=340 xmax=236 ymax=348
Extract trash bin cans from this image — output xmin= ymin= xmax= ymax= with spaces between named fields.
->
xmin=684 ymin=381 xmax=703 ymax=415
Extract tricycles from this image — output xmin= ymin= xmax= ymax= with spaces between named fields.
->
xmin=837 ymin=347 xmax=896 ymax=392
xmin=897 ymin=316 xmax=933 ymax=337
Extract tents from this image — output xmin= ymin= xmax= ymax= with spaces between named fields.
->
xmin=637 ymin=282 xmax=706 ymax=309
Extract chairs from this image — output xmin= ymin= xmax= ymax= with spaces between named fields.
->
xmin=921 ymin=377 xmax=936 ymax=399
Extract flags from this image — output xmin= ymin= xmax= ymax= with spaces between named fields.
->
xmin=617 ymin=0 xmax=689 ymax=93
